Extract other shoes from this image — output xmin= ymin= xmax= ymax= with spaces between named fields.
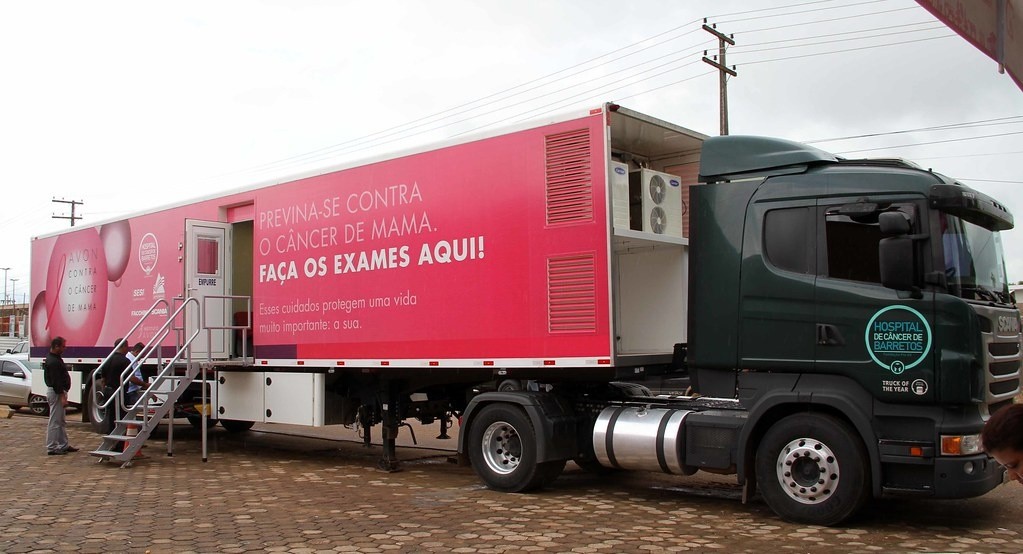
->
xmin=62 ymin=446 xmax=80 ymax=452
xmin=47 ymin=448 xmax=67 ymax=455
xmin=141 ymin=445 xmax=148 ymax=448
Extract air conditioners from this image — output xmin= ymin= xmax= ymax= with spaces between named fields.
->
xmin=628 ymin=167 xmax=682 ymax=237
xmin=562 ymin=155 xmax=630 ymax=230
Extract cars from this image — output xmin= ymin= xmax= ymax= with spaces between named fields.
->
xmin=0 ymin=352 xmax=65 ymax=415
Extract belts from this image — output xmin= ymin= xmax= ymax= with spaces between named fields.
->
xmin=105 ymin=385 xmax=112 ymax=389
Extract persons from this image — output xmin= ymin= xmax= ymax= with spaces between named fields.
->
xmin=125 ymin=342 xmax=157 ymax=410
xmin=43 ymin=337 xmax=80 ymax=454
xmin=101 ymin=338 xmax=154 ymax=452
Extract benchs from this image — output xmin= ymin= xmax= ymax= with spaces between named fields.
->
xmin=234 ymin=311 xmax=253 ymax=358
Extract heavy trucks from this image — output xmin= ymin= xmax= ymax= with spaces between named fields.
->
xmin=28 ymin=102 xmax=1023 ymax=526
